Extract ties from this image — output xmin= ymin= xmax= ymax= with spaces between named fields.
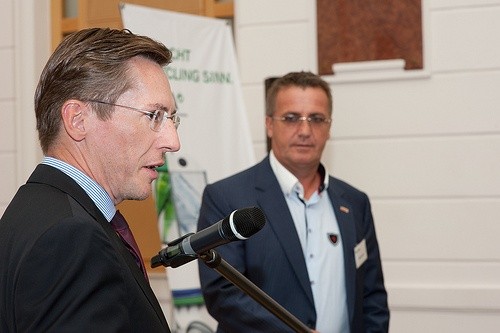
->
xmin=109 ymin=208 xmax=149 ymax=290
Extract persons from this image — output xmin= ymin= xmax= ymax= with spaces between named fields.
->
xmin=0 ymin=27 xmax=181 ymax=333
xmin=196 ymin=70 xmax=389 ymax=333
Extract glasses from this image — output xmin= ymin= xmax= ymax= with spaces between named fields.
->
xmin=80 ymin=98 xmax=180 ymax=132
xmin=272 ymin=113 xmax=331 ymax=124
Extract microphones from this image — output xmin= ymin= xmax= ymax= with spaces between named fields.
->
xmin=150 ymin=207 xmax=266 ymax=269
